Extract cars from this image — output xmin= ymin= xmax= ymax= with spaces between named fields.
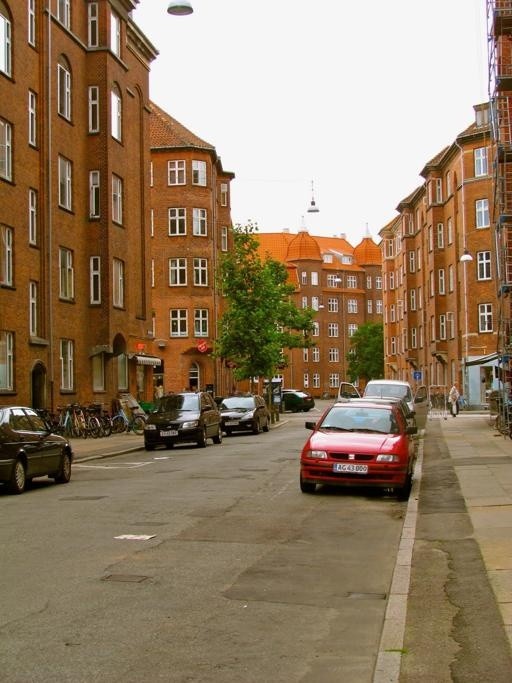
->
xmin=146 ymin=388 xmax=316 ymax=449
xmin=299 ymin=378 xmax=430 ymax=500
xmin=0 ymin=404 xmax=74 ymax=492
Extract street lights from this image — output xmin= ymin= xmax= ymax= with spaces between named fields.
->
xmin=459 ymin=248 xmax=474 ymax=404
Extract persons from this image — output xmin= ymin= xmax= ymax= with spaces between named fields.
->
xmin=448 ymin=381 xmax=461 ymax=403
xmin=449 ymin=395 xmax=459 ymax=418
xmin=153 ymin=383 xmax=240 ymax=408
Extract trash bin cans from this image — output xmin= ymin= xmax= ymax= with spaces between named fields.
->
xmin=323 ymin=392 xmax=329 ymax=399
xmin=141 ymin=403 xmax=156 ymax=414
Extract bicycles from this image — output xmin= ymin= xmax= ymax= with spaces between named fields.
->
xmin=36 ymin=400 xmax=147 ymax=439
